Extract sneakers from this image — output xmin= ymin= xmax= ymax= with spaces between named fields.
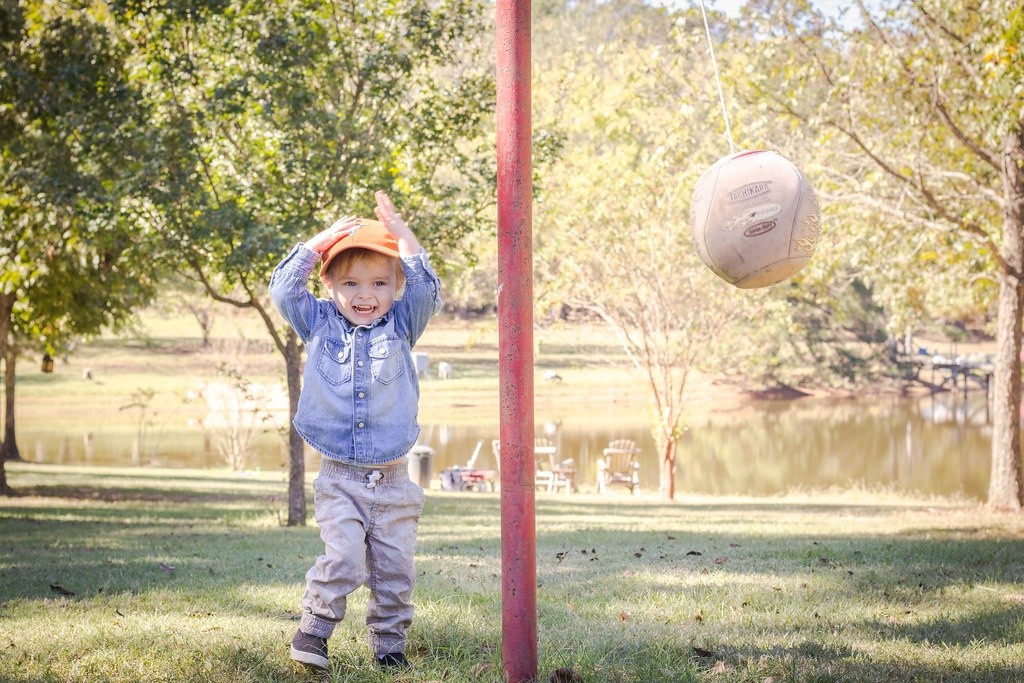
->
xmin=371 ymin=653 xmax=428 ymax=680
xmin=290 ymin=627 xmax=329 ymax=669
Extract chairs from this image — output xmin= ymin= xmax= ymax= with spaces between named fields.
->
xmin=595 ymin=439 xmax=640 ymax=496
xmin=535 ymin=438 xmax=580 ymax=495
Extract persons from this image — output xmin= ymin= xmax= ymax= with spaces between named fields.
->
xmin=267 ymin=190 xmax=443 ymax=670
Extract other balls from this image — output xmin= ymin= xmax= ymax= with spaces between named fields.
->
xmin=687 ymin=148 xmax=822 ymax=287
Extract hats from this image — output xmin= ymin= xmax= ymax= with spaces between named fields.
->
xmin=320 ymin=218 xmax=399 ymax=276
